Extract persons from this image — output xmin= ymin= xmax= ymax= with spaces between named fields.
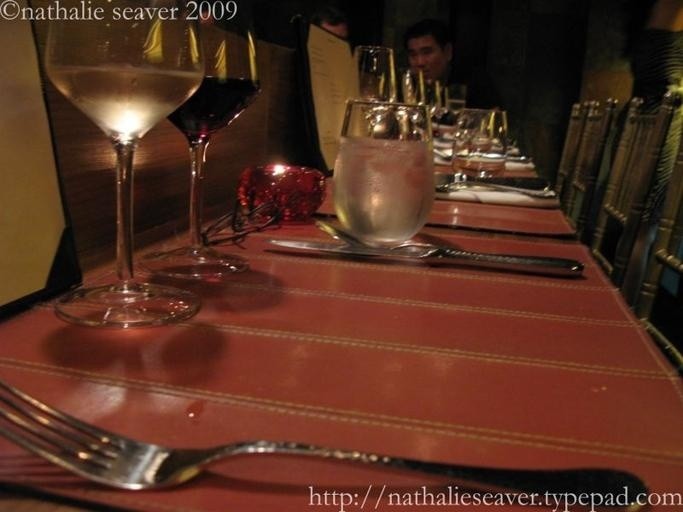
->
xmin=616 ymin=1 xmax=681 ymax=309
xmin=308 ymin=6 xmax=349 ymax=41
xmin=396 ymin=19 xmax=498 ymax=107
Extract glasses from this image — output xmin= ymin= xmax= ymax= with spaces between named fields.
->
xmin=202 ymin=202 xmax=281 ymax=243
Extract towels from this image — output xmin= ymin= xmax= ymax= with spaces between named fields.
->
xmin=435 ymin=189 xmax=561 ymax=208
xmin=432 ymin=122 xmax=536 ymax=172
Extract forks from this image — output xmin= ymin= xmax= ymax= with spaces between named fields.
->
xmin=0 ymin=379 xmax=652 ymax=510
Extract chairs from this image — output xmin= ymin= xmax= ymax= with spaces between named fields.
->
xmin=555 ymin=95 xmax=683 ymax=382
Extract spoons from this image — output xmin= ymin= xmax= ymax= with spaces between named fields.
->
xmin=311 ymin=216 xmax=580 ymax=268
xmin=433 ymin=179 xmax=558 ymax=202
xmin=430 ymin=146 xmax=534 ymax=165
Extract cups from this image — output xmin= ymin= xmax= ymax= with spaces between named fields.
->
xmin=330 ymin=45 xmax=509 ymax=250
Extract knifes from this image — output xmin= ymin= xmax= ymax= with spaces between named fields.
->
xmin=270 ymin=237 xmax=585 ymax=279
xmin=432 ymin=170 xmax=550 ymax=191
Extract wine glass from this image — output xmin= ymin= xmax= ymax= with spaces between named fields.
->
xmin=42 ymin=1 xmax=207 ymax=330
xmin=136 ymin=20 xmax=268 ymax=282
